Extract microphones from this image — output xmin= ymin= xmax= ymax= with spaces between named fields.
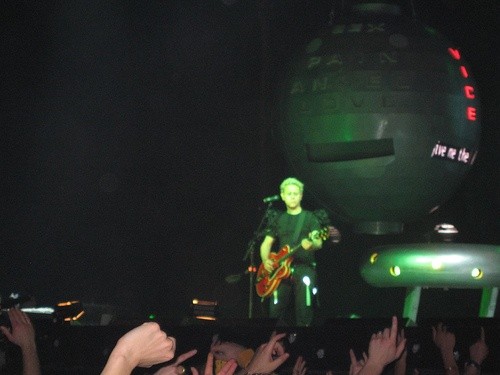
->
xmin=262 ymin=194 xmax=283 ymax=203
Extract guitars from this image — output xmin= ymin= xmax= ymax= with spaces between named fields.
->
xmin=254 ymin=227 xmax=332 ymax=299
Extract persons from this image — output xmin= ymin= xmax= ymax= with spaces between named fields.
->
xmin=259 ymin=178 xmax=324 ymax=327
xmin=0 ymin=303 xmax=499 ymax=375
